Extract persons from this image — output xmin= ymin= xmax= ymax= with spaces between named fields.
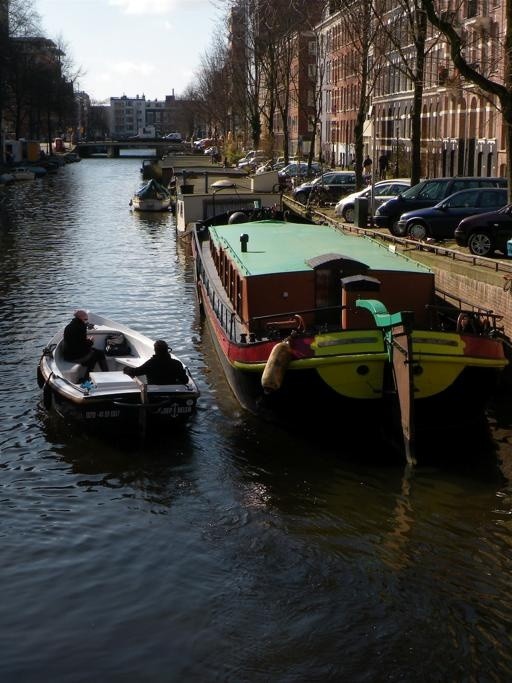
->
xmin=40 ymin=150 xmax=45 ymax=160
xmin=125 ymin=340 xmax=189 ymax=385
xmin=364 ymin=155 xmax=372 ymax=175
xmin=352 ymin=159 xmax=356 ymax=171
xmin=6 ymin=149 xmax=15 ymax=169
xmin=64 ymin=310 xmax=108 ymax=382
xmin=379 ymin=151 xmax=387 ymax=181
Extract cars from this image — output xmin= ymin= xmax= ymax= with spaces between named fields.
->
xmin=455 ymin=204 xmax=512 ymax=257
xmin=504 ymin=236 xmax=512 ymax=260
xmin=159 ymin=129 xmax=430 ymax=225
xmin=398 ymin=184 xmax=509 ymax=245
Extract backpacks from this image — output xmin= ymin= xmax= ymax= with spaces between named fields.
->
xmin=105 ymin=333 xmax=131 ymax=356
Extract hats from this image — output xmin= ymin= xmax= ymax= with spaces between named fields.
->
xmin=74 ymin=311 xmax=88 ymax=320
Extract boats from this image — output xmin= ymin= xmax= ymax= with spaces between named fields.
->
xmin=139 ymin=158 xmax=155 ymax=180
xmin=181 ymin=177 xmax=512 ymax=463
xmin=129 ymin=177 xmax=174 ymax=212
xmin=32 ymin=307 xmax=204 ymax=432
xmin=5 ymin=152 xmax=81 ymax=180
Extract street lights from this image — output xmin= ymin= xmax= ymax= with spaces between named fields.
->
xmin=68 ymin=126 xmax=73 ymax=149
xmin=394 ymin=114 xmax=403 ymax=165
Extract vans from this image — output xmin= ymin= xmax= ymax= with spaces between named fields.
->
xmin=372 ymin=174 xmax=509 ymax=239
